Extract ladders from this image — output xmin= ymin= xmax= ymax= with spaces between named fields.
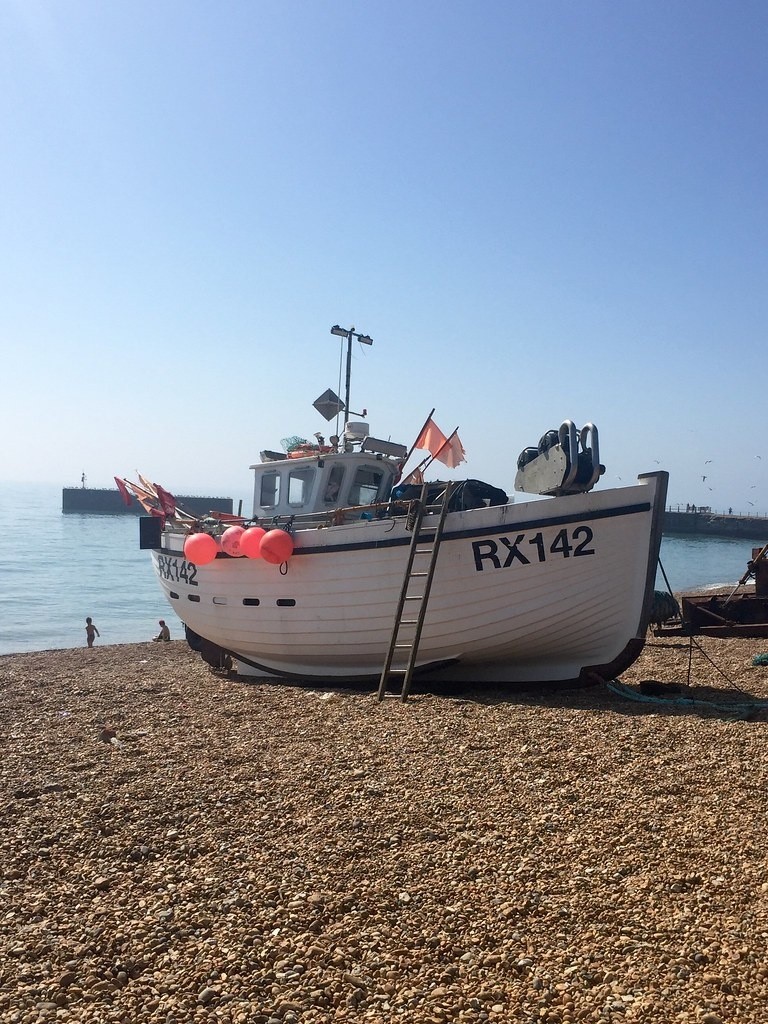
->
xmin=376 ymin=482 xmax=451 ymax=703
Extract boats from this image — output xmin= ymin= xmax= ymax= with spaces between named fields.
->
xmin=135 ymin=327 xmax=670 ymax=696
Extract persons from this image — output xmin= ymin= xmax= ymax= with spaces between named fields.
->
xmin=692 ymin=504 xmax=696 ymax=512
xmin=81 ymin=472 xmax=85 ymax=488
xmin=85 ymin=617 xmax=100 ymax=647
xmin=153 ymin=621 xmax=170 ymax=642
xmin=686 ymin=503 xmax=690 ymax=512
xmin=729 ymin=506 xmax=732 ymax=514
